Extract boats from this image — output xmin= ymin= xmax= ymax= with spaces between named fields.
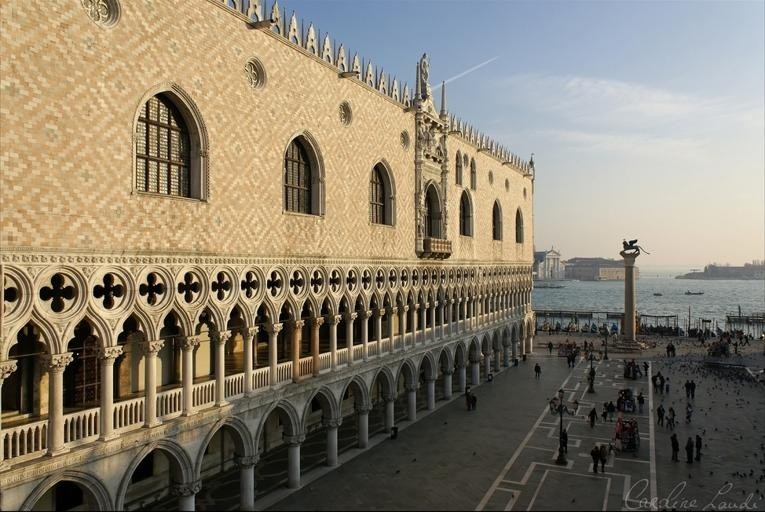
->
xmin=683 ymin=288 xmax=706 ymax=296
xmin=652 ymin=291 xmax=662 ymax=296
xmin=534 ymin=282 xmax=565 ymax=289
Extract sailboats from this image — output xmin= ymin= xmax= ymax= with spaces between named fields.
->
xmin=535 ymin=305 xmax=765 ymax=339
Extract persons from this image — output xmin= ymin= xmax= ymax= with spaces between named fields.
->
xmin=467 ymin=393 xmax=477 ymax=410
xmin=419 ymin=50 xmax=431 ymax=83
xmin=533 ymin=321 xmax=765 ymax=474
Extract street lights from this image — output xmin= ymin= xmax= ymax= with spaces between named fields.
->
xmin=586 ymin=343 xmax=596 ymax=394
xmin=547 ymin=387 xmax=578 ymax=466
xmin=601 ymin=324 xmax=610 ymax=360
